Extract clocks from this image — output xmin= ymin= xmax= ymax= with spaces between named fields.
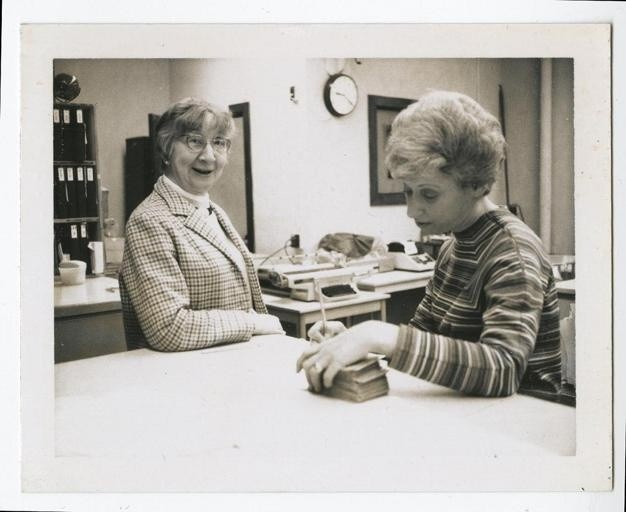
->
xmin=324 ymin=74 xmax=359 ymax=118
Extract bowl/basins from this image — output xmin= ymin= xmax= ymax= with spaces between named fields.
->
xmin=58 ymin=259 xmax=87 ymax=287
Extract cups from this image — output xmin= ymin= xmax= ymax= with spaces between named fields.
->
xmin=87 ymin=241 xmax=106 ymax=274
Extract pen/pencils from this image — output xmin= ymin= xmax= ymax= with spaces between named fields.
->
xmin=317 ymin=280 xmax=326 ymax=337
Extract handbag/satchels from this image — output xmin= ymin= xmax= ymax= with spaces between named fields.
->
xmin=318 ymin=232 xmax=373 ymax=259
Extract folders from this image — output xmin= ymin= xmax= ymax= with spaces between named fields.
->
xmin=53 ymin=106 xmax=96 ymax=275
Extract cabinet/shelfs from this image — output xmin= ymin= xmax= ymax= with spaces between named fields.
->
xmin=54 ymin=103 xmax=104 ymax=276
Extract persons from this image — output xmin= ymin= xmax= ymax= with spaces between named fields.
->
xmin=296 ymin=89 xmax=565 ymax=404
xmin=117 ymin=97 xmax=286 ymax=351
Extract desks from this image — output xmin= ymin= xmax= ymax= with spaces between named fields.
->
xmin=261 ymin=255 xmax=438 ymax=340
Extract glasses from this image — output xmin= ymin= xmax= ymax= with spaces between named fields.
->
xmin=177 ymin=134 xmax=231 ymax=154
